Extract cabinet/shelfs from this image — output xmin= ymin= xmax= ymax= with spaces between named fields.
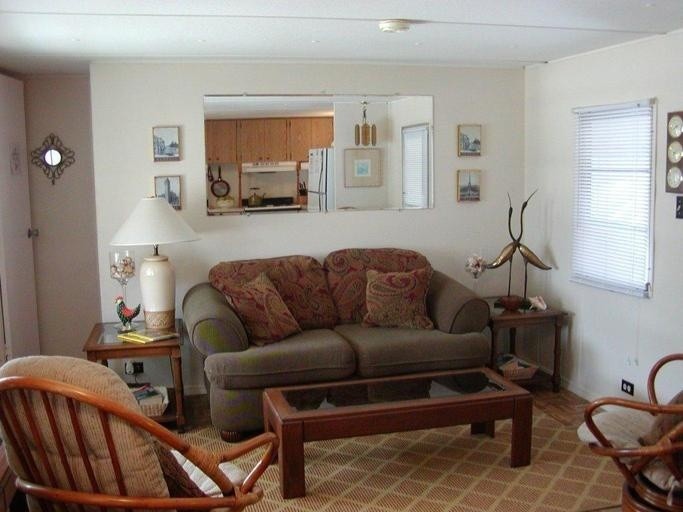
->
xmin=204 ymin=119 xmax=236 ymax=164
xmin=288 ymin=117 xmax=332 ymax=161
xmin=237 ymin=118 xmax=287 ymax=162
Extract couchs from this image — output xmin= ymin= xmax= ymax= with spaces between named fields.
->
xmin=180 ymin=270 xmax=492 ymax=445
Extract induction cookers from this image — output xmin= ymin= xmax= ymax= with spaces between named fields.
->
xmin=243 ymin=199 xmax=300 ymax=212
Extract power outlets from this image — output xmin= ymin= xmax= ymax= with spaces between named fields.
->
xmin=124 ymin=361 xmax=143 ymax=375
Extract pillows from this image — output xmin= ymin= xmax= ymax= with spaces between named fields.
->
xmin=323 ymin=247 xmax=437 ymax=328
xmin=149 ymin=438 xmax=213 ymax=512
xmin=637 ymin=384 xmax=683 ymax=486
xmin=206 ymin=253 xmax=339 ymax=331
xmin=219 ymin=272 xmax=301 ymax=347
xmin=360 ymin=267 xmax=438 ymax=331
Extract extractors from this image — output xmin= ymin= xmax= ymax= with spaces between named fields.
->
xmin=241 ymin=161 xmax=296 ymax=175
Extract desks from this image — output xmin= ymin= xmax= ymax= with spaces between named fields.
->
xmin=81 ymin=318 xmax=186 ymax=435
xmin=482 ymin=294 xmax=571 ymax=393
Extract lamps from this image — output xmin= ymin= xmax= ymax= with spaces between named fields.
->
xmin=107 ymin=196 xmax=200 ymax=330
xmin=353 ymin=101 xmax=377 ymax=146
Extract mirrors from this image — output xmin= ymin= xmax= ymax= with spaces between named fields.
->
xmin=28 ymin=132 xmax=76 ymax=187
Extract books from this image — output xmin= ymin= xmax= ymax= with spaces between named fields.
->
xmin=116 ymin=330 xmax=179 ymax=344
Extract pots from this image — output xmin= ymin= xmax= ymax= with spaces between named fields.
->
xmin=210 ymin=166 xmax=230 ymax=197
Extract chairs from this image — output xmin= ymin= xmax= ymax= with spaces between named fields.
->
xmin=0 ymin=355 xmax=280 ymax=512
xmin=575 ymin=352 xmax=683 ymax=512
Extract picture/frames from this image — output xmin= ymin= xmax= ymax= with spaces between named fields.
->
xmin=343 ymin=149 xmax=382 ymax=188
xmin=152 ymin=174 xmax=182 ymax=211
xmin=151 ymin=124 xmax=182 ymax=162
xmin=456 ymin=123 xmax=481 ymax=158
xmin=455 ymin=169 xmax=481 ymax=203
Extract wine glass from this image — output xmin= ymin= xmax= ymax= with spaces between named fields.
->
xmin=107 ymin=249 xmax=139 ymax=329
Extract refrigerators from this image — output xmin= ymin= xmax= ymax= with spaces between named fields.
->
xmin=306 ymin=148 xmax=334 ymax=210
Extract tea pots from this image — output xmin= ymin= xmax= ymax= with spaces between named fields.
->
xmin=247 ymin=188 xmax=266 ymax=207
